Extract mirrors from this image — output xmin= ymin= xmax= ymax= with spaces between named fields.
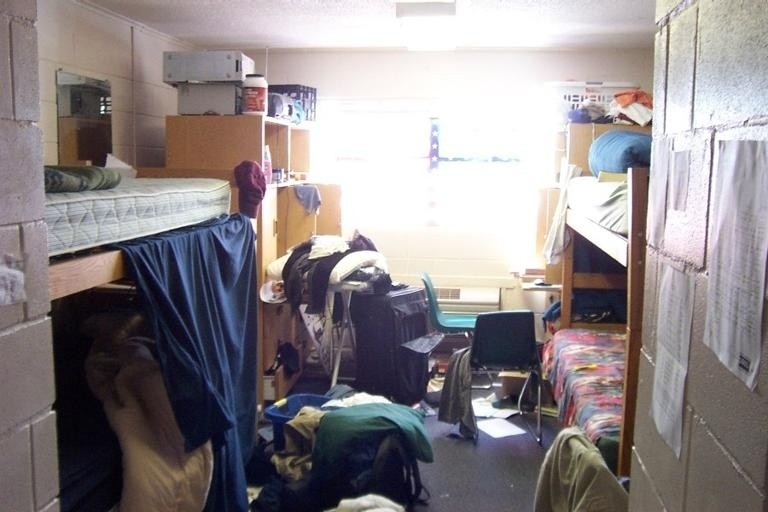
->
xmin=55 ymin=66 xmax=113 ymax=170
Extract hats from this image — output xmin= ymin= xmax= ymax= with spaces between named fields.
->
xmin=233 ymin=159 xmax=269 ymax=220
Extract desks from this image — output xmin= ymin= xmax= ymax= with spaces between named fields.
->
xmin=281 ymin=278 xmax=393 ymax=387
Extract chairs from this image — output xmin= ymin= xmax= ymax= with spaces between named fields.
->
xmin=456 ymin=309 xmax=544 ymax=448
xmin=420 ymin=271 xmax=494 ymax=390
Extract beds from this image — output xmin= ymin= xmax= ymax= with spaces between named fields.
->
xmin=41 ymin=161 xmax=264 ymax=512
xmin=541 ymin=166 xmax=652 ymax=488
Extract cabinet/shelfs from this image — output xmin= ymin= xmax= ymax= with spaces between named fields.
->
xmin=163 ymin=115 xmax=344 ymax=403
xmin=536 ymin=120 xmax=651 ymax=331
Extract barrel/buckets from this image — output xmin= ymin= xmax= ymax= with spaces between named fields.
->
xmin=241 ymin=74 xmax=268 ymax=116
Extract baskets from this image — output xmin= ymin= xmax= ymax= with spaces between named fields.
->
xmin=266 ymin=84 xmax=316 ymax=122
xmin=545 ymin=81 xmax=639 ymax=121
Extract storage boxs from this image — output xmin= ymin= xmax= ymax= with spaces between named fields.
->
xmin=265 ymin=393 xmax=332 ymax=453
xmin=160 ymin=43 xmax=255 ymax=114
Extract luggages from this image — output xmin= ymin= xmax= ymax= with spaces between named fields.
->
xmin=351 ymin=280 xmax=448 ymax=406
